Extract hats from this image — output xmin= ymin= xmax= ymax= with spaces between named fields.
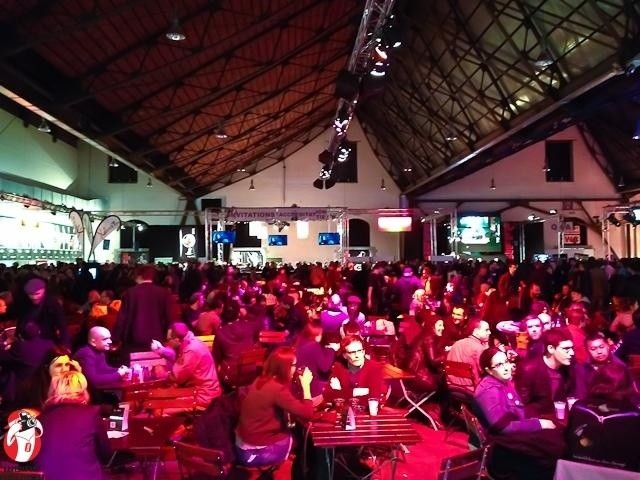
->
xmin=25 ymin=279 xmax=46 ymax=294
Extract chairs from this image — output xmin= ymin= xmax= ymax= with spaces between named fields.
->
xmin=0 ymin=281 xmax=640 ymax=480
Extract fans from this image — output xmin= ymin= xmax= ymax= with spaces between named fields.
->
xmin=182 ymin=234 xmax=196 ymax=256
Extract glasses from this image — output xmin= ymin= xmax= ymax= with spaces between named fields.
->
xmin=490 ymin=359 xmax=509 ymax=370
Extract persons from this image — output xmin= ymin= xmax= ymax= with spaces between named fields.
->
xmin=0 ymin=253 xmax=640 ymax=479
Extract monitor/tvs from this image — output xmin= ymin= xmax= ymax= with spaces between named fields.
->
xmin=212 ymin=231 xmax=238 ymax=244
xmin=269 ymin=235 xmax=287 ymax=246
xmin=319 ymin=233 xmax=340 ymax=245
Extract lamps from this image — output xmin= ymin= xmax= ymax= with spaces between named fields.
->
xmin=443 ymin=125 xmax=553 ymax=189
xmin=312 ymin=13 xmax=413 ymax=190
xmin=532 ymin=46 xmax=559 ymax=71
xmin=211 ymin=124 xmax=260 ymax=191
xmin=163 ymin=12 xmax=190 ymax=42
xmin=38 ymin=119 xmax=154 ymax=190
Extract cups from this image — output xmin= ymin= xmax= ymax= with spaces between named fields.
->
xmin=368 ymin=401 xmax=379 ymax=417
xmin=554 ymin=398 xmax=577 ymax=420
